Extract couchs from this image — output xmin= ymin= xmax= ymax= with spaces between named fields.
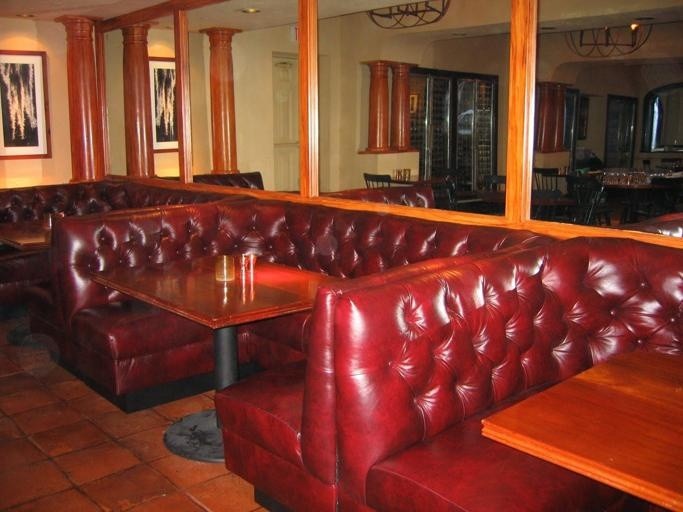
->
xmin=1 ymin=182 xmax=683 ymax=512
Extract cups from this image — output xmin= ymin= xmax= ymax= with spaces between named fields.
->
xmin=215 ymin=254 xmax=236 ymax=282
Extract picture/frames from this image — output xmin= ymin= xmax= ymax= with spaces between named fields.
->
xmin=1 ymin=50 xmax=51 ymax=160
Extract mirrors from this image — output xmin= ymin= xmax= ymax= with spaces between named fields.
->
xmin=92 ymin=0 xmax=683 ymax=245
